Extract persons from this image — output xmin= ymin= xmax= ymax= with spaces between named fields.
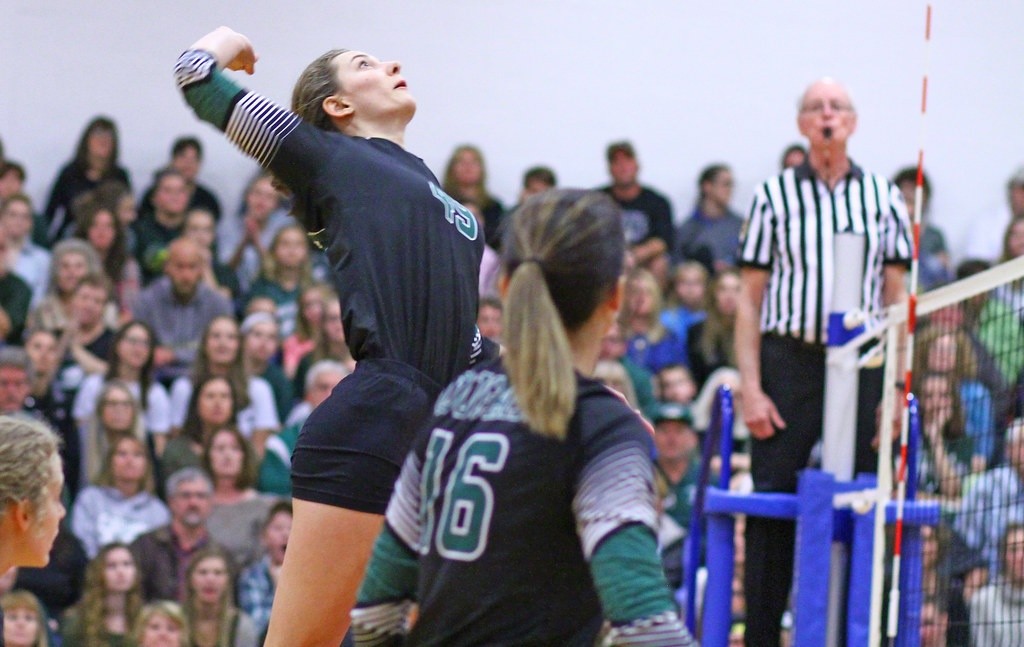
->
xmin=173 ymin=26 xmax=506 ymax=647
xmin=0 ymin=74 xmax=1024 ymax=646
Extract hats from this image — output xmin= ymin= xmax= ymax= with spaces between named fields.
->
xmin=608 ymin=142 xmax=634 ymax=159
xmin=651 ymin=401 xmax=690 ymax=422
